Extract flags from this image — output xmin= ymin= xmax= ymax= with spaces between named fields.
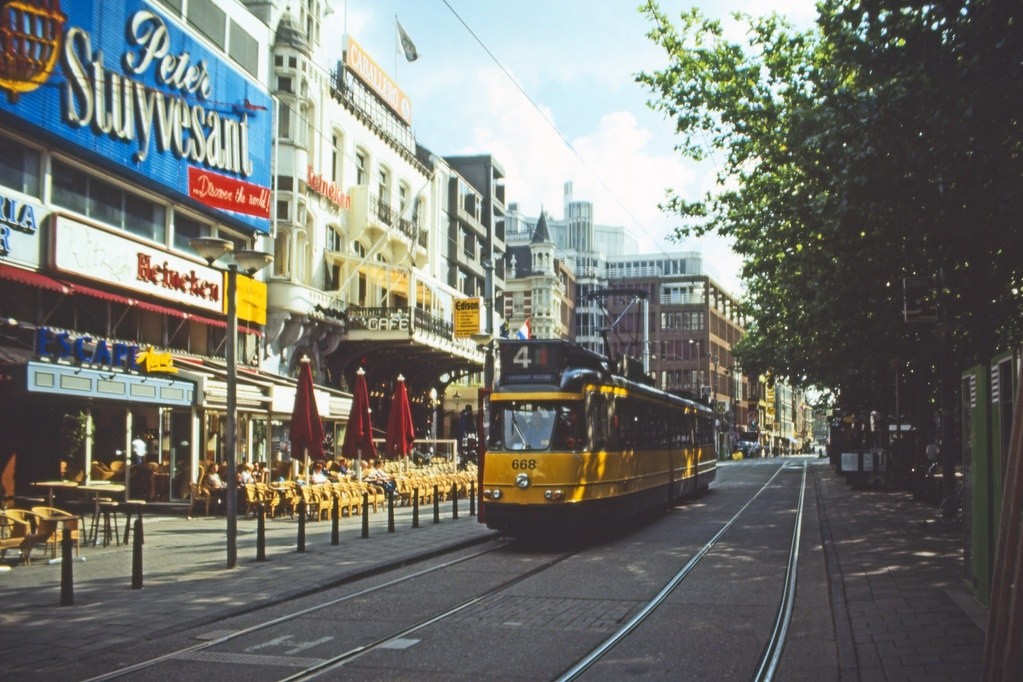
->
xmin=516 ymin=320 xmax=531 ymax=340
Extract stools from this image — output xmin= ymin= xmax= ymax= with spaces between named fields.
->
xmin=89 ymin=498 xmax=112 ymax=540
xmin=123 ymin=500 xmax=146 ymax=544
xmin=93 ymin=502 xmax=119 ymax=547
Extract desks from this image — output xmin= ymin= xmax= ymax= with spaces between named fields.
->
xmin=29 ymin=482 xmax=78 ymax=507
xmin=77 ymin=484 xmax=126 ymax=545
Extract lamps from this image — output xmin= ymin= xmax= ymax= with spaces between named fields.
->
xmin=300 ymin=296 xmax=346 ymax=320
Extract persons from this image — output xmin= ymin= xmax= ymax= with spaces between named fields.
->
xmin=524 ymin=412 xmax=556 ymax=449
xmin=310 ymin=459 xmax=330 ymax=485
xmin=338 ymin=458 xmax=399 ymax=497
xmin=132 ymin=434 xmax=147 ymax=465
xmin=207 ymin=462 xmax=261 ymax=517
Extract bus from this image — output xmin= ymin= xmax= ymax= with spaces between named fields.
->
xmin=477 ymin=327 xmax=721 ymax=545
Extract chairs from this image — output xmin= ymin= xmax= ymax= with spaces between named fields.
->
xmin=0 ymin=506 xmax=82 ymax=567
xmin=185 ymin=457 xmax=479 ymax=522
xmin=70 ymin=458 xmax=170 ymax=501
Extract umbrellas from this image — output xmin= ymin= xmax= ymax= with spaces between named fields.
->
xmin=288 ymin=353 xmax=327 ymax=515
xmin=384 ymin=373 xmax=416 ymax=478
xmin=341 ymin=367 xmax=377 ymax=487
